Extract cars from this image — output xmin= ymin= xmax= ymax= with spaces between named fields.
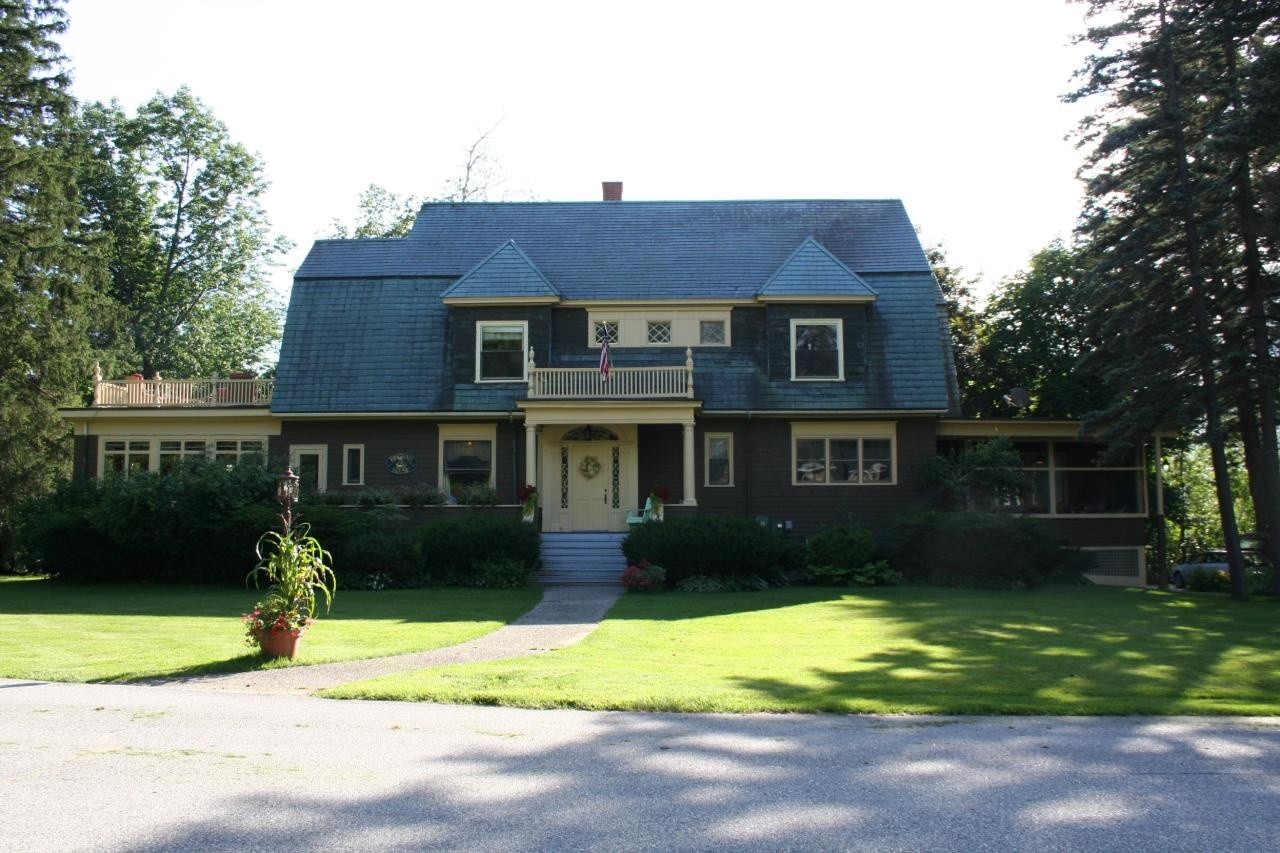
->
xmin=1172 ymin=547 xmax=1275 ymax=597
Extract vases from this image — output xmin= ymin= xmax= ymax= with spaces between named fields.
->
xmin=254 ymin=628 xmax=299 ymax=662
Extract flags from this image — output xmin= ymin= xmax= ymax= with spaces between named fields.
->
xmin=600 ymin=335 xmax=610 ymax=383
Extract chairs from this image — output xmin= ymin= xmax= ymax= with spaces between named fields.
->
xmin=626 ymin=496 xmax=663 ymax=528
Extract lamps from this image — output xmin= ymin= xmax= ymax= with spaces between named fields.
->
xmin=583 ymin=424 xmax=593 ymax=443
xmin=271 ymin=464 xmax=303 ymax=505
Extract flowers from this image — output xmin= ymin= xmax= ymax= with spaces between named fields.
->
xmin=240 ymin=519 xmax=336 ymax=646
